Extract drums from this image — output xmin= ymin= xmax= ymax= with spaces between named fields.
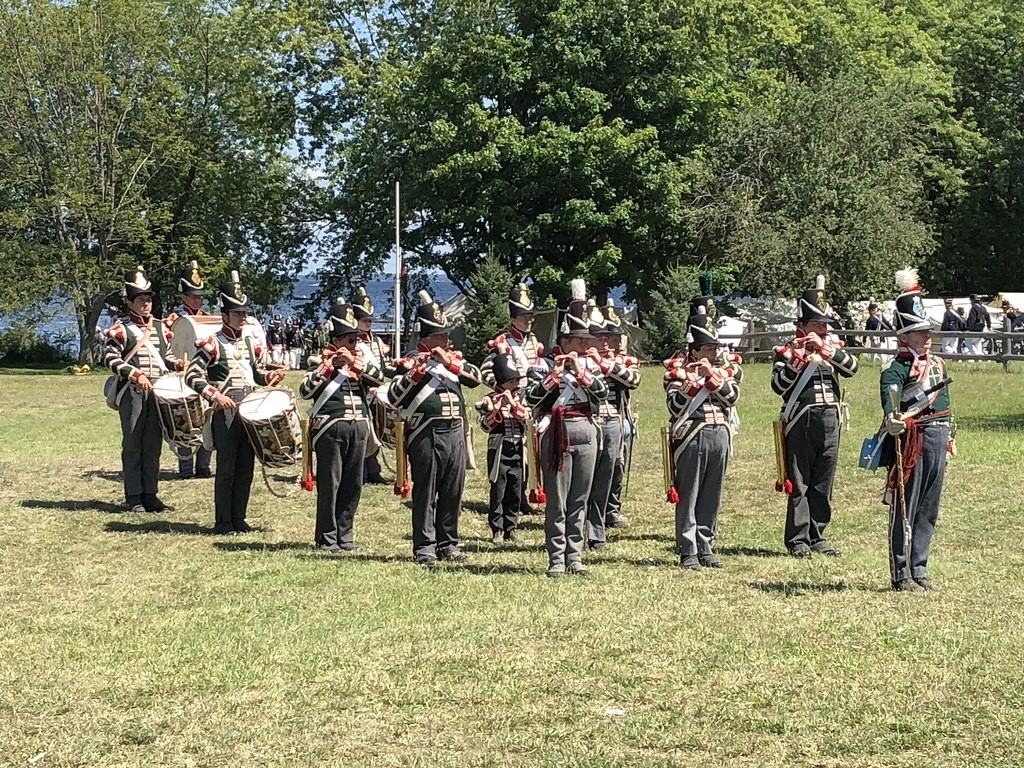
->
xmin=151 ymin=370 xmax=210 ymax=448
xmin=238 ymin=384 xmax=304 ymax=466
xmin=168 ymin=315 xmax=267 ymax=376
xmin=374 ymin=380 xmax=407 ymax=450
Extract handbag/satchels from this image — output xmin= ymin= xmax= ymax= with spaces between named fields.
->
xmin=104 ymin=374 xmax=119 ymax=412
xmin=202 ymin=407 xmax=215 ymax=452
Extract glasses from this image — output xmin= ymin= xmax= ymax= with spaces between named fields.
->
xmin=343 ymin=338 xmax=358 ymax=343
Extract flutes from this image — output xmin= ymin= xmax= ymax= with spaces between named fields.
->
xmin=419 ymin=344 xmax=454 ymax=363
xmin=690 ymin=358 xmax=723 ymax=369
xmin=724 ymin=350 xmax=743 ymax=354
xmin=323 ymin=348 xmax=361 ymax=362
xmin=596 ymin=348 xmax=617 ymax=353
xmin=554 ymin=352 xmax=589 ymax=365
xmin=792 ymin=333 xmax=829 ymax=346
xmin=878 ymin=377 xmax=954 ymax=465
xmin=495 ymin=387 xmax=522 ymax=402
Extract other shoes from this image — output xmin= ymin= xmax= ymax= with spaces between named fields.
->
xmin=892 ymin=577 xmax=939 ymax=591
xmin=315 ymin=543 xmax=361 ymax=554
xmin=547 ymin=569 xmax=595 ymax=580
xmin=214 ymin=526 xmax=259 ymax=535
xmin=369 ymin=475 xmax=390 ymax=485
xmin=589 ymin=541 xmax=605 ymax=550
xmin=492 ymin=530 xmax=523 ymax=543
xmin=122 ymin=499 xmax=175 ymax=512
xmin=680 ymin=554 xmax=724 ymax=571
xmin=185 ymin=469 xmax=216 ymax=479
xmin=610 ymin=521 xmax=628 ymax=529
xmin=793 ymin=548 xmax=842 ymax=558
xmin=416 ymin=550 xmax=468 ymax=566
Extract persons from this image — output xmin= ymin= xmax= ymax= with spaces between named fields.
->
xmin=90 ymin=314 xmax=334 ymax=371
xmin=101 ymin=263 xmax=192 ymax=511
xmin=299 ymin=297 xmax=388 ymax=552
xmin=349 ymin=285 xmax=413 ymax=484
xmin=662 ymin=294 xmax=746 ymax=556
xmin=524 ymin=299 xmax=610 ymax=577
xmin=386 ymin=288 xmax=481 ymax=560
xmin=567 ymin=278 xmax=641 ymax=549
xmin=685 ymin=275 xmax=728 ymax=349
xmin=770 ymin=274 xmax=859 ymax=558
xmin=479 ymin=279 xmax=545 ymax=515
xmin=184 ymin=271 xmax=285 ymax=532
xmin=863 ymin=288 xmax=1024 ymax=362
xmin=475 ymin=352 xmax=532 ymax=543
xmin=160 ymin=259 xmax=215 ymax=478
xmin=870 ymin=266 xmax=958 ymax=595
xmin=663 ymin=314 xmax=741 ymax=570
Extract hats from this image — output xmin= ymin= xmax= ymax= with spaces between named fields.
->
xmin=486 ymin=340 xmax=527 ymax=387
xmin=178 ymin=260 xmax=207 ymax=295
xmin=261 ymin=268 xmax=1013 ymax=338
xmin=122 ymin=265 xmax=156 ymax=298
xmin=219 ymin=270 xmax=251 ymax=311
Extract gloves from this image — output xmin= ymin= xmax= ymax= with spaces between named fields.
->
xmin=884 ymin=413 xmax=905 ymax=435
xmin=946 ymin=439 xmax=956 ymax=465
